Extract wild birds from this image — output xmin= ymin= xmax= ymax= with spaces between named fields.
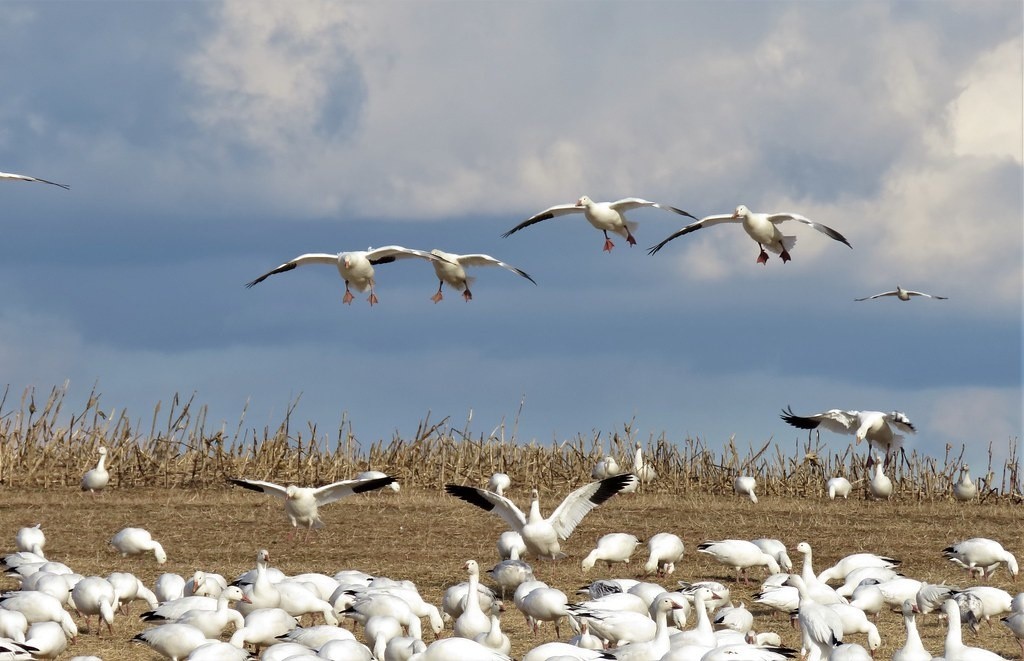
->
xmin=422 ymin=248 xmax=536 ymax=304
xmin=779 ymin=403 xmax=919 ymax=471
xmin=952 ymin=464 xmax=976 ymax=501
xmin=590 ymin=457 xmax=618 ymax=480
xmin=734 ymin=477 xmax=759 ymax=504
xmin=225 ymin=473 xmax=405 ymax=543
xmin=614 ymin=474 xmax=641 ymax=496
xmin=853 ymin=286 xmax=949 ymax=301
xmin=645 ymin=205 xmax=854 ymax=266
xmin=580 ymin=532 xmax=644 ymax=576
xmin=80 ymin=447 xmax=110 ymax=502
xmin=641 ymin=533 xmax=686 ymax=584
xmin=244 ymin=245 xmax=458 ymax=305
xmin=498 ymin=530 xmax=527 ymax=560
xmin=490 ymin=474 xmax=511 ymax=496
xmin=2 ymin=537 xmax=1024 ymax=661
xmin=824 ymin=477 xmax=853 ymax=501
xmin=499 ymin=195 xmax=698 ymax=254
xmin=108 ymin=528 xmax=167 ymax=565
xmin=443 ymin=473 xmax=633 ymax=575
xmin=632 ymin=441 xmax=655 ymax=486
xmin=871 ymin=456 xmax=894 ymax=501
xmin=15 ymin=524 xmax=47 ymax=558
